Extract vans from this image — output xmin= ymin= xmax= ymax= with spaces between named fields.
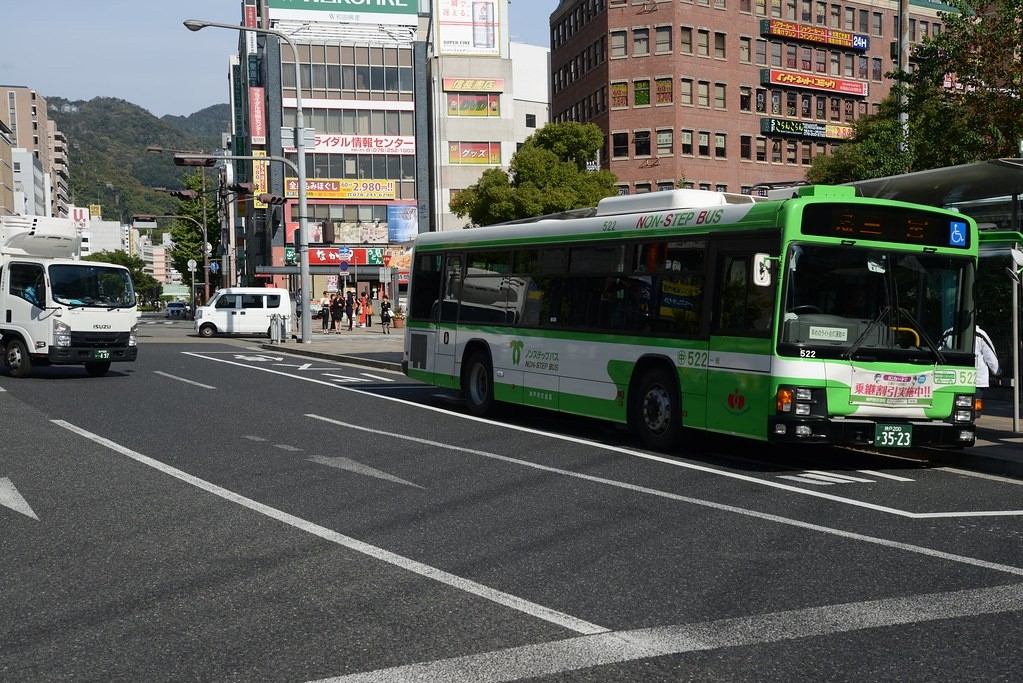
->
xmin=193 ymin=287 xmax=291 ymax=338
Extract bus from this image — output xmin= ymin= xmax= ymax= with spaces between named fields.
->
xmin=400 ymin=188 xmax=1023 ymax=464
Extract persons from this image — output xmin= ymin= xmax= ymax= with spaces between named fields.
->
xmin=321 ymin=290 xmax=330 ymax=334
xmin=296 ymin=288 xmax=302 ymax=332
xmin=357 ymin=292 xmax=366 ymax=328
xmin=330 ymin=291 xmax=357 ymax=334
xmin=381 ymin=295 xmax=392 ymax=334
xmin=937 ymin=323 xmax=1002 ymax=441
xmin=366 ymin=292 xmax=374 ymax=329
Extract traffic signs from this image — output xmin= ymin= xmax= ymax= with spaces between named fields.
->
xmin=339 ymin=246 xmax=350 ymax=261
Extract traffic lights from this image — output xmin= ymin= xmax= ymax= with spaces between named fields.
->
xmin=227 ymin=182 xmax=255 ymax=195
xmin=170 ymin=190 xmax=198 ymax=201
xmin=258 ymin=193 xmax=288 ymax=206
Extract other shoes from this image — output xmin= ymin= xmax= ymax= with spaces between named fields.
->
xmin=320 ymin=322 xmax=391 ymax=334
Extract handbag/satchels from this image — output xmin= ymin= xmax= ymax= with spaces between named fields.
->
xmin=387 ymin=309 xmax=394 ymax=317
xmin=366 ymin=304 xmax=373 ymax=316
xmin=357 ymin=305 xmax=362 ymax=314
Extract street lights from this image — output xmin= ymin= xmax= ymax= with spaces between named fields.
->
xmin=184 ymin=19 xmax=314 ymax=343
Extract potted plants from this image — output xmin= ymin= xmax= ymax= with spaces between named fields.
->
xmin=392 ymin=308 xmax=404 ymax=328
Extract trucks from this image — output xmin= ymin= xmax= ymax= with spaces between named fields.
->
xmin=431 ymin=266 xmax=542 ymax=328
xmin=0 ymin=215 xmax=140 ymax=379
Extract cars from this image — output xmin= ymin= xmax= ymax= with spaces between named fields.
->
xmin=165 ymin=302 xmax=188 ymax=318
